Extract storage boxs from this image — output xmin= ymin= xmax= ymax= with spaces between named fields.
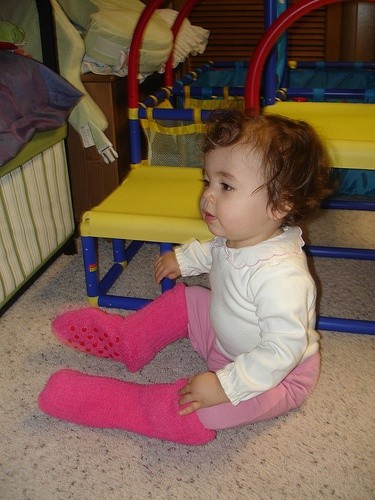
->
xmin=0 ymin=123 xmax=76 ymax=319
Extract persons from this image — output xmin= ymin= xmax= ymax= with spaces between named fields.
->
xmin=38 ymin=106 xmax=341 ymax=446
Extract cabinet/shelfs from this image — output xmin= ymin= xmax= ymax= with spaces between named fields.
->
xmin=65 ymin=72 xmax=160 ymax=244
